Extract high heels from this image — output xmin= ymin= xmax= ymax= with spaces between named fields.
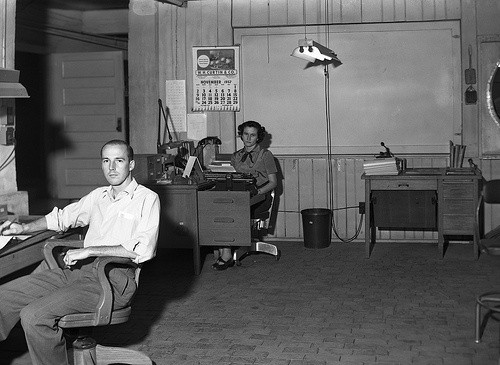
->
xmin=212 ymin=256 xmax=234 ymax=270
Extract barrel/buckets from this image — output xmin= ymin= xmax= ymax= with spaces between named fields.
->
xmin=301 ymin=208 xmax=332 ymax=248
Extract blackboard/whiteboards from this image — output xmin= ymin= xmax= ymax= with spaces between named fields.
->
xmin=233 ymin=21 xmax=462 ymax=153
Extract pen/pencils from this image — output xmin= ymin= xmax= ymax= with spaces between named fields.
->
xmin=0 ymin=218 xmax=17 ymax=236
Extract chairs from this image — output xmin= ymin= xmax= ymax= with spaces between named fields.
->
xmin=41 ymin=239 xmax=153 ymax=365
xmin=233 ymin=157 xmax=282 ymax=265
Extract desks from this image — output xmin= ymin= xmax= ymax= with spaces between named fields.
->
xmin=360 ymin=168 xmax=482 ymax=259
xmin=0 ymin=224 xmax=87 ymax=280
xmin=138 ymin=172 xmax=255 ymax=276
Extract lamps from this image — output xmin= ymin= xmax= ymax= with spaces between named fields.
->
xmin=290 ymin=0 xmax=343 ymax=65
xmin=156 ymin=99 xmax=182 ymax=153
xmin=197 ymin=137 xmax=223 ymax=159
xmin=0 ymin=67 xmax=31 ymax=98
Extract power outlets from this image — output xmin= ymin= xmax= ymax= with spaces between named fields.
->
xmin=0 ymin=126 xmax=15 ymax=144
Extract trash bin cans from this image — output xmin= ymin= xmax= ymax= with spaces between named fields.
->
xmin=301 ymin=208 xmax=332 ymax=249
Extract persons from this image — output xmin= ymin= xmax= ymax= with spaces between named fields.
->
xmin=210 ymin=121 xmax=278 ymax=272
xmin=0 ymin=139 xmax=161 ymax=365
